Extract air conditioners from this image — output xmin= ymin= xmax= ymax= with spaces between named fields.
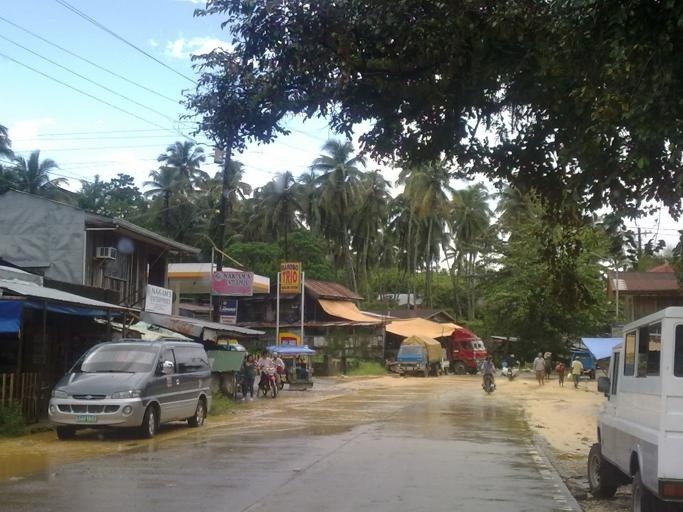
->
xmin=95 ymin=246 xmax=117 ymax=260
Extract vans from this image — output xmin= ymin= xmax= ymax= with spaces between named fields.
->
xmin=45 ymin=338 xmax=212 ymax=441
xmin=585 ymin=305 xmax=683 ymax=512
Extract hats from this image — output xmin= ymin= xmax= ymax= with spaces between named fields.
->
xmin=575 ymin=357 xmax=579 ymax=359
xmin=538 ymin=353 xmax=542 ymax=357
xmin=273 ymin=352 xmax=277 ymax=357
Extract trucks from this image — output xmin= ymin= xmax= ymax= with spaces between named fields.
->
xmin=447 ymin=327 xmax=487 ymax=375
xmin=396 ymin=334 xmax=444 ymax=378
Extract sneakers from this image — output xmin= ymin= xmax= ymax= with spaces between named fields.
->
xmin=241 ymin=397 xmax=254 ymax=401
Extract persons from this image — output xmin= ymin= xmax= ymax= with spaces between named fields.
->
xmin=481 ymin=356 xmax=496 ymax=388
xmin=533 ymin=352 xmax=545 ymax=386
xmin=570 ymin=355 xmax=584 ymax=389
xmin=241 ymin=354 xmax=256 ymax=401
xmin=271 ymin=352 xmax=285 ymax=370
xmin=558 ymin=362 xmax=565 ymax=387
xmin=544 ymin=352 xmax=552 ymax=380
xmin=256 ymin=352 xmax=274 ymax=397
